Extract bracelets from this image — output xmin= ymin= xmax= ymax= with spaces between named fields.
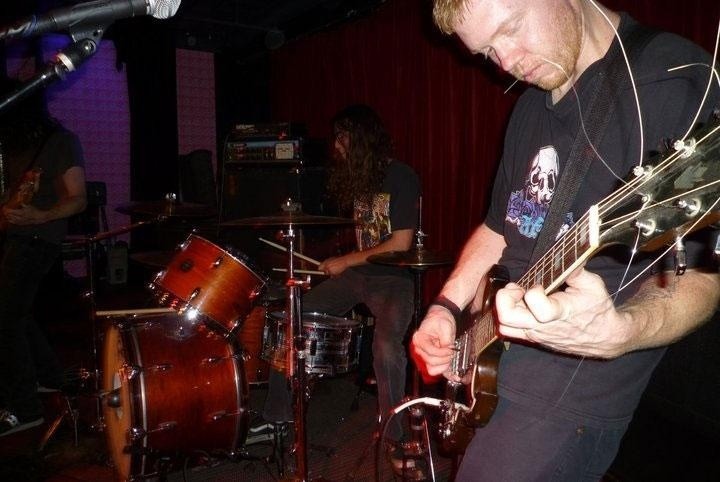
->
xmin=432 ymin=294 xmax=464 ymax=322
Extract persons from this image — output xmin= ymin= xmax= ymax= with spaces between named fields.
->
xmin=413 ymin=0 xmax=719 ymax=482
xmin=244 ymin=104 xmax=420 ymax=469
xmin=0 ymin=73 xmax=89 ymax=439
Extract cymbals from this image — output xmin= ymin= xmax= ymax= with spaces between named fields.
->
xmin=212 ymin=203 xmax=358 ymax=226
xmin=129 ymin=249 xmax=175 ymax=267
xmin=368 ymin=241 xmax=459 ymax=266
xmin=113 ymin=192 xmax=218 ymax=220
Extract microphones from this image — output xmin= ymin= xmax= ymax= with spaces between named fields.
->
xmin=0 ymin=0 xmax=182 ymax=41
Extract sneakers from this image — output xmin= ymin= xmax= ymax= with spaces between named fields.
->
xmin=385 ymin=442 xmax=415 ymax=468
xmin=243 ymin=421 xmax=290 ymax=446
xmin=0 ymin=405 xmax=45 ymax=438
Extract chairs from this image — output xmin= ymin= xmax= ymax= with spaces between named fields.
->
xmin=58 ymin=180 xmax=112 ymax=252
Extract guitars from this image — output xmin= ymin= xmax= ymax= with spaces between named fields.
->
xmin=424 ymin=117 xmax=720 ymax=455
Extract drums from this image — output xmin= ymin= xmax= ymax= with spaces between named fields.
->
xmin=261 ymin=307 xmax=364 ymax=377
xmin=148 ymin=229 xmax=268 ymax=341
xmin=92 ymin=307 xmax=251 ymax=481
xmin=235 ymin=294 xmax=280 ymax=385
